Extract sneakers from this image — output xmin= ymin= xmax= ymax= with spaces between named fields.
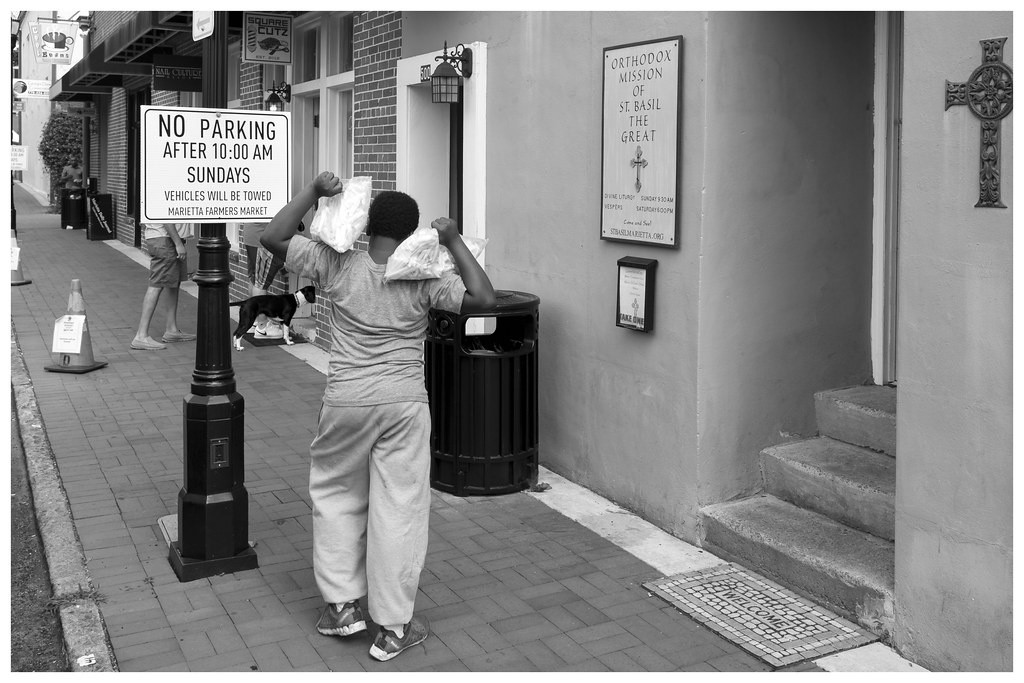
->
xmin=253 ymin=322 xmax=283 ymax=339
xmin=246 ymin=320 xmax=258 ymax=333
xmin=369 ymin=615 xmax=430 ymax=661
xmin=315 ymin=599 xmax=370 ymax=636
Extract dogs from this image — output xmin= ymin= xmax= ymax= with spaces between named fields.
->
xmin=230 ymin=286 xmax=315 ymax=350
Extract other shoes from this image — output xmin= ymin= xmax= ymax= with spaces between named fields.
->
xmin=162 ymin=329 xmax=196 ymax=342
xmin=130 ymin=336 xmax=166 ymax=350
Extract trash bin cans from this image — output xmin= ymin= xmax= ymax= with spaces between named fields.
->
xmin=60 ymin=188 xmax=86 ymax=230
xmin=424 ymin=287 xmax=541 ymax=496
xmin=87 ymin=192 xmax=113 ymax=241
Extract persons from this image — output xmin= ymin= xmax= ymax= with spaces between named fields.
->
xmin=61 ymin=156 xmax=83 ymax=188
xmin=243 ymin=223 xmax=284 ymax=340
xmin=260 ymin=171 xmax=496 ymax=661
xmin=131 ymin=222 xmax=196 ymax=351
